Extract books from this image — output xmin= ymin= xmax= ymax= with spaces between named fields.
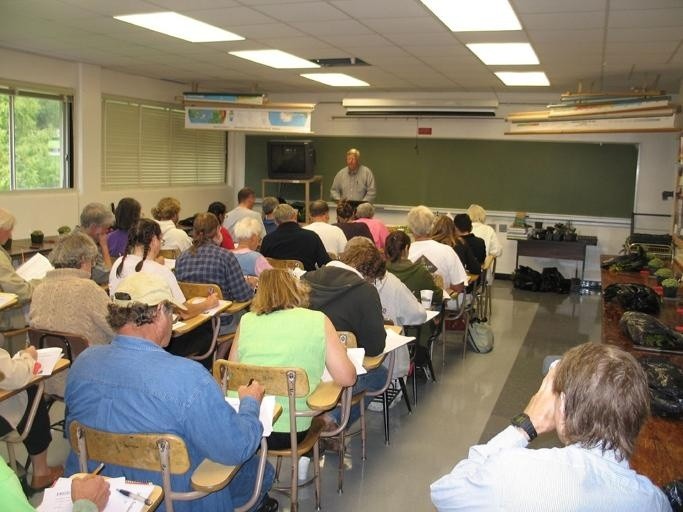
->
xmin=506 ymin=225 xmax=533 ymax=241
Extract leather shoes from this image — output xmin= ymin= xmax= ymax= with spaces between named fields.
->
xmin=29 ymin=464 xmax=64 ymax=493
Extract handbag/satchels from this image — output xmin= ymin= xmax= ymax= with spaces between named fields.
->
xmin=539 ymin=267 xmax=566 ymax=291
xmin=512 ymin=265 xmax=539 ymax=291
xmin=467 ymin=315 xmax=493 ymax=354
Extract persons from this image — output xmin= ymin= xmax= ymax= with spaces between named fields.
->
xmin=332 ymin=202 xmax=375 ymax=244
xmin=64 ymin=271 xmax=279 ymax=512
xmin=329 ymin=149 xmax=375 ymax=223
xmin=207 ymin=201 xmax=235 ymax=249
xmin=0 ymin=454 xmax=111 ymax=512
xmin=302 ymin=199 xmax=347 ymax=260
xmin=351 ymin=202 xmax=392 ymax=250
xmin=454 ymin=213 xmax=486 ymax=294
xmin=70 ymin=202 xmax=115 ymax=285
xmin=0 ymin=208 xmax=43 ymax=356
xmin=430 ymin=342 xmax=673 ymax=512
xmin=223 ymin=188 xmax=266 ymax=246
xmin=260 ymin=204 xmax=332 ymax=271
xmin=27 ymin=230 xmax=118 ymax=398
xmin=107 ymin=197 xmax=142 ymax=257
xmin=299 ymin=247 xmax=389 ymax=454
xmin=432 ymin=216 xmax=481 ymax=275
xmin=108 ymin=218 xmax=219 ymax=356
xmin=226 ymin=216 xmax=274 ymax=278
xmin=151 ymin=197 xmax=194 ymax=270
xmin=0 ymin=345 xmax=64 ymax=492
xmin=223 ymin=269 xmax=358 ymax=481
xmin=406 ymin=205 xmax=469 ymax=301
xmin=344 ymin=236 xmax=427 ymax=379
xmin=383 ymin=231 xmax=443 ymax=383
xmin=261 ymin=196 xmax=279 ymax=234
xmin=174 ymin=212 xmax=255 ymax=360
xmin=466 ymin=204 xmax=502 ymax=287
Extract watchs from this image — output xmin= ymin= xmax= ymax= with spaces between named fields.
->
xmin=511 ymin=413 xmax=538 ymax=442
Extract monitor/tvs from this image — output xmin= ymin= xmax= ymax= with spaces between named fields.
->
xmin=267 ymin=139 xmax=315 ymax=178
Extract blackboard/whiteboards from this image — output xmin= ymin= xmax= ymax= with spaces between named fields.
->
xmin=243 ymin=133 xmax=641 ymax=227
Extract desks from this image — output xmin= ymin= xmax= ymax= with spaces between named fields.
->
xmin=263 ymin=174 xmax=323 ymax=226
xmin=599 ymin=253 xmax=683 ymax=512
xmin=7 ymin=236 xmax=58 ymax=262
xmin=507 ymin=235 xmax=598 ymax=281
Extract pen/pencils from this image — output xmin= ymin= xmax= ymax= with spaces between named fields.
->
xmin=87 ymin=462 xmax=105 ymax=481
xmin=25 ymin=337 xmax=29 ymax=349
xmin=247 ymin=377 xmax=254 ymax=387
xmin=207 ymin=284 xmax=215 ymax=298
xmin=115 ymin=487 xmax=152 ymax=507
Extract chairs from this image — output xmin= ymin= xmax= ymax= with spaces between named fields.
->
xmin=68 ymin=402 xmax=282 ymax=512
xmin=0 ymin=357 xmax=72 ymax=503
xmin=212 ymin=358 xmax=352 ymax=512
xmin=26 ymin=327 xmax=90 ymax=433
xmin=265 ymin=257 xmax=305 ymax=272
xmin=299 ymin=506 xmax=300 ymax=508
xmin=433 ymin=275 xmax=479 ymax=366
xmin=0 ymin=290 xmax=32 ymax=356
xmin=170 ymin=295 xmax=232 ymax=359
xmin=481 ymin=254 xmax=495 ymax=318
xmin=385 ymin=317 xmax=436 ymax=414
xmin=177 ymin=282 xmax=254 ymax=345
xmin=156 ymin=248 xmax=181 ymax=260
xmin=335 ymin=324 xmax=404 ymax=462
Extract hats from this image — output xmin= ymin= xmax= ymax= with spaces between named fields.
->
xmin=113 ymin=271 xmax=188 ymax=312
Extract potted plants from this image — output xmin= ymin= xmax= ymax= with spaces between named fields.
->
xmin=647 ymin=252 xmax=655 ymax=261
xmin=654 ymin=267 xmax=673 ymax=287
xmin=647 ymin=257 xmax=664 ymax=275
xmin=661 ymin=278 xmax=679 ymax=299
